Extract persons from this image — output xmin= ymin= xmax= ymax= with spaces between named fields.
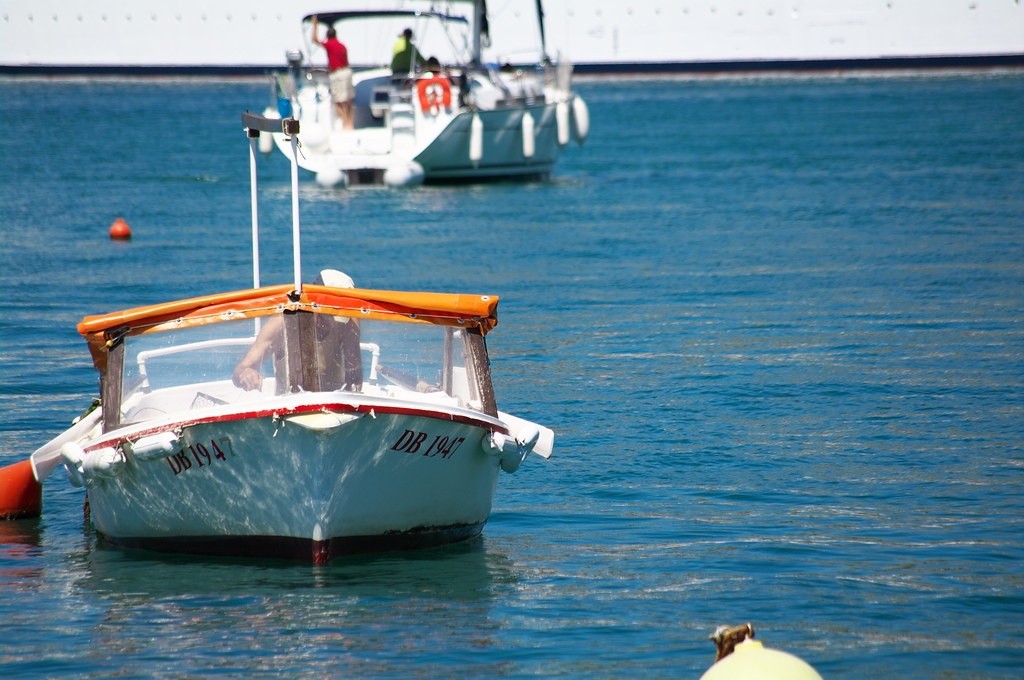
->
xmin=311 ymin=13 xmax=356 ymax=132
xmin=232 ymin=269 xmax=364 ymax=396
xmin=391 ymin=27 xmax=428 ymax=103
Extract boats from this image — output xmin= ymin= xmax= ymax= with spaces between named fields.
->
xmin=262 ymin=0 xmax=575 ymax=185
xmin=59 ymin=111 xmax=555 ymax=569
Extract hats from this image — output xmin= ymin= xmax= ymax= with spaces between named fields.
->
xmin=313 ymin=268 xmax=356 ymax=324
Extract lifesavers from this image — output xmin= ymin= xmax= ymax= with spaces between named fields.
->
xmin=419 ymin=74 xmax=451 ymax=110
